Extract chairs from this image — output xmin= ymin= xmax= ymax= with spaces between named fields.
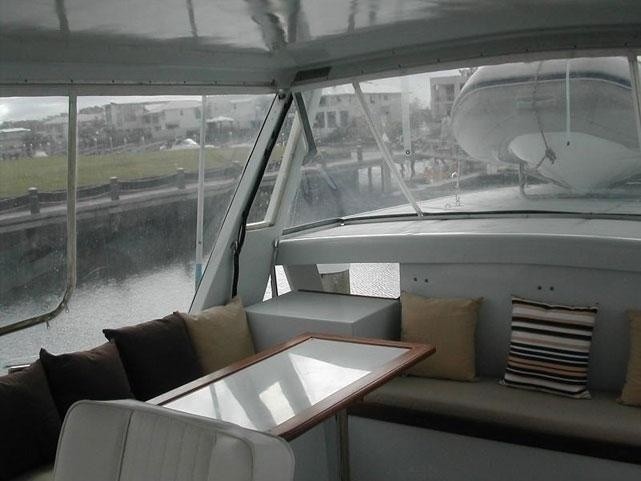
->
xmin=52 ymin=399 xmax=297 ymax=481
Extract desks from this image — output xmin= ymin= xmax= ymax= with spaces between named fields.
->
xmin=144 ymin=332 xmax=437 ymax=481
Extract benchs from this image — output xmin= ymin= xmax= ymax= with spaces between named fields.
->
xmin=345 ymin=374 xmax=641 ymax=481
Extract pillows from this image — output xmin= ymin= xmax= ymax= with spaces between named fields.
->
xmin=173 ymin=293 xmax=255 ymax=377
xmin=495 ymin=293 xmax=602 ymax=401
xmin=103 ymin=309 xmax=204 ymax=402
xmin=400 ymin=289 xmax=484 ymax=383
xmin=0 ymin=358 xmax=62 ymax=481
xmin=39 ymin=337 xmax=137 ymax=423
xmin=615 ymin=302 xmax=641 ymax=408
